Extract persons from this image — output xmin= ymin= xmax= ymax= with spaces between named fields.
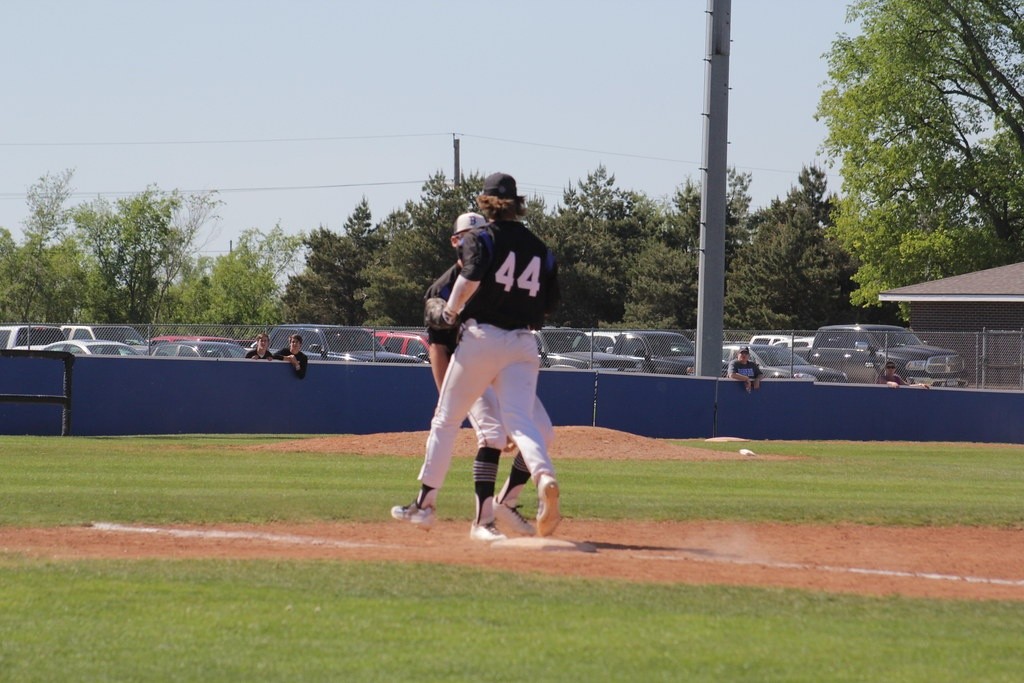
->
xmin=727 ymin=346 xmax=766 ymax=393
xmin=246 ymin=335 xmax=273 ymax=361
xmin=880 ymin=361 xmax=930 ymax=390
xmin=390 ymin=173 xmax=561 ymax=543
xmin=274 ymin=334 xmax=307 ymax=379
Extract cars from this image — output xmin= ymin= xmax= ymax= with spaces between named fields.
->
xmin=0 ymin=322 xmax=971 ymax=389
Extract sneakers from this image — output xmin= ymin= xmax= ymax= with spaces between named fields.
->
xmin=392 ymin=501 xmax=434 ymax=531
xmin=470 ymin=521 xmax=508 ymax=541
xmin=493 ymin=495 xmax=536 ymax=536
xmin=538 ymin=475 xmax=561 ymax=536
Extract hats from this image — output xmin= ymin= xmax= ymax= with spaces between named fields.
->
xmin=484 ymin=172 xmax=518 ymax=198
xmin=453 ymin=212 xmax=489 ymax=233
xmin=738 ymin=346 xmax=749 ymax=354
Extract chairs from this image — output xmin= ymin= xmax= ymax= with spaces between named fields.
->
xmin=100 ymin=347 xmax=121 ymax=355
xmin=408 ymin=344 xmax=420 ymax=357
xmin=156 ymin=351 xmax=169 ymax=356
